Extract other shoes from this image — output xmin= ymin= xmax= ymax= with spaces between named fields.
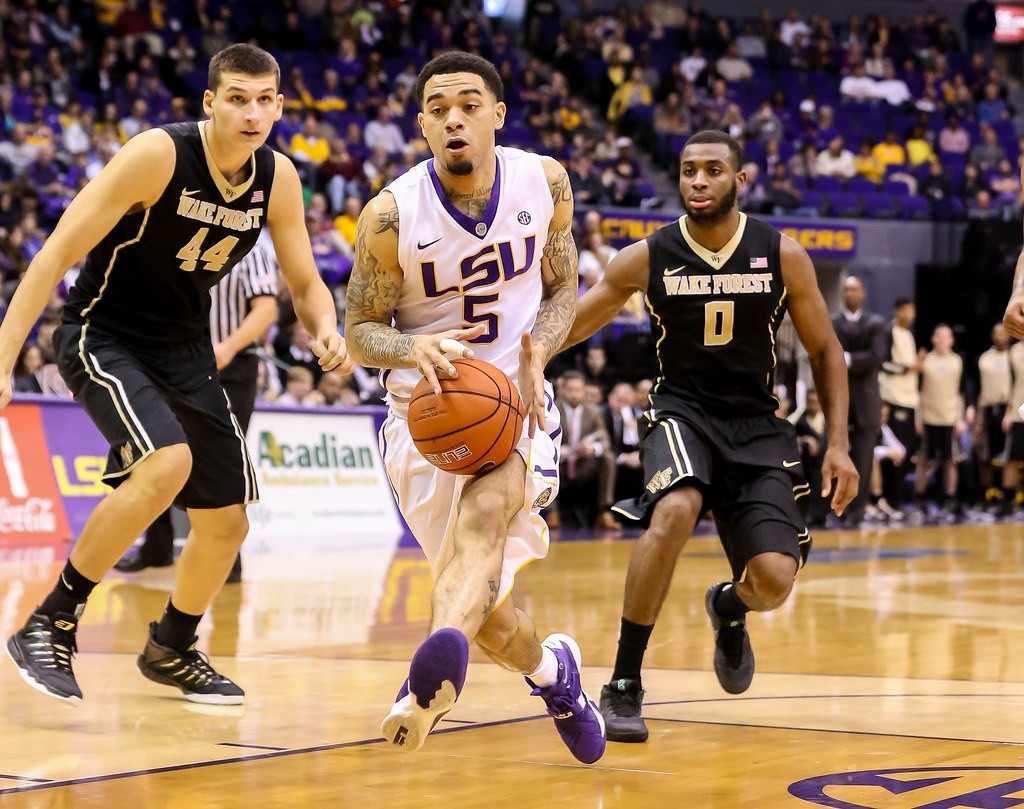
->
xmin=545 ymin=512 xmax=562 ymax=530
xmin=864 ymin=494 xmax=1024 ymax=527
xmin=596 ymin=514 xmax=625 ymax=531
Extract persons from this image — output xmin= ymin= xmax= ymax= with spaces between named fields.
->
xmin=545 ymin=345 xmax=652 ymax=531
xmin=606 ymin=0 xmax=1024 ymax=218
xmin=0 ymin=43 xmax=349 ymax=705
xmin=772 ymin=274 xmax=907 ymax=528
xmin=345 ymin=50 xmax=607 ymax=764
xmin=878 ymin=239 xmax=1024 ymax=515
xmin=552 ymin=132 xmax=861 ymax=743
xmin=0 ymin=0 xmax=643 ymax=407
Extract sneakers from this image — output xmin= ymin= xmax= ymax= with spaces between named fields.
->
xmin=599 ymin=677 xmax=649 ymax=744
xmin=4 ymin=608 xmax=83 ymax=704
xmin=377 ymin=626 xmax=470 ymax=751
xmin=705 ymin=581 xmax=757 ymax=695
xmin=134 ymin=619 xmax=247 ymax=708
xmin=519 ymin=633 xmax=608 ymax=765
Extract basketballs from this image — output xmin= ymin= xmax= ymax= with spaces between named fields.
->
xmin=407 ymin=357 xmax=526 ymax=477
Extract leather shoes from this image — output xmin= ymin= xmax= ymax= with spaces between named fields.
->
xmin=111 ymin=544 xmax=177 ymax=573
xmin=224 ymin=550 xmax=243 ymax=585
